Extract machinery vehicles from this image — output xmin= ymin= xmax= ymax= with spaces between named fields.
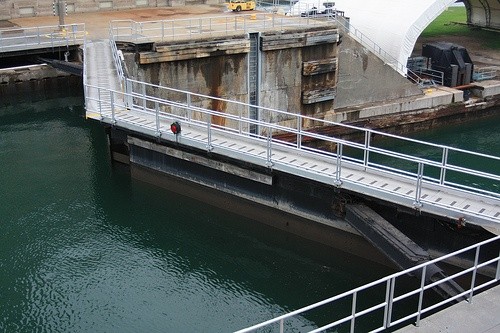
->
xmin=228 ymin=0 xmax=255 ymax=12
